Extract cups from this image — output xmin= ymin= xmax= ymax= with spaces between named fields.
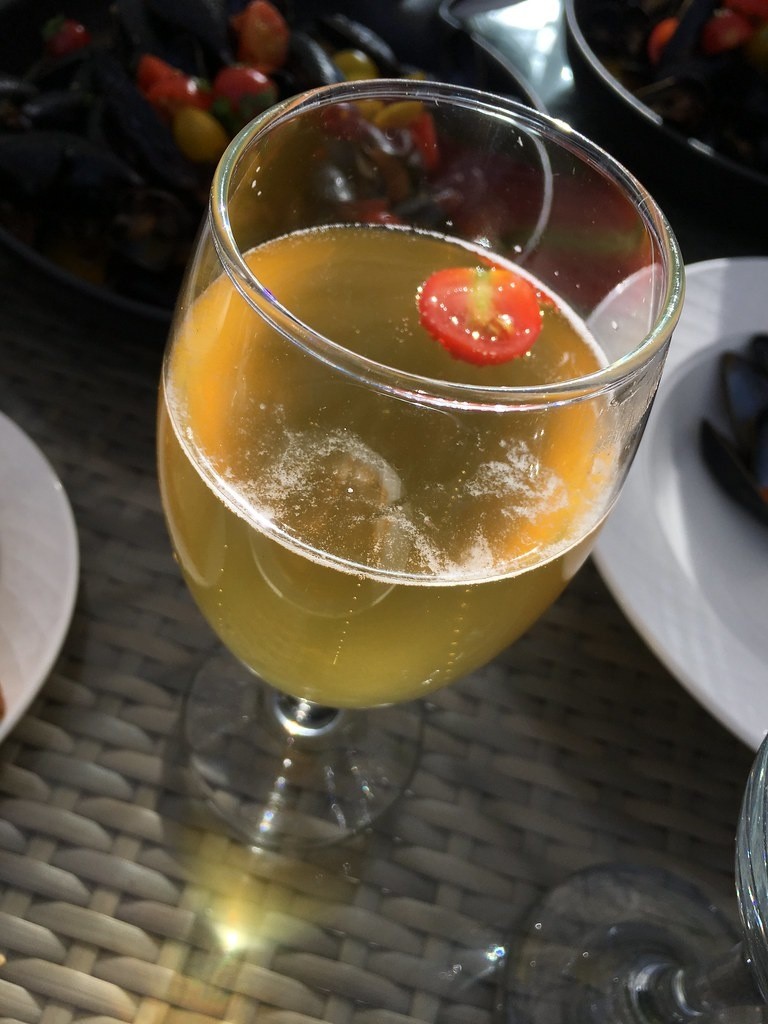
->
xmin=495 ymin=734 xmax=768 ymax=1024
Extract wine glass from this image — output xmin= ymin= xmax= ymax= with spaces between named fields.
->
xmin=157 ymin=79 xmax=685 ymax=851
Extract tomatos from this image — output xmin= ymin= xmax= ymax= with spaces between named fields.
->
xmin=419 ymin=267 xmax=542 ymax=364
xmin=644 ymin=0 xmax=768 ymax=69
xmin=45 ymin=0 xmax=445 ymax=229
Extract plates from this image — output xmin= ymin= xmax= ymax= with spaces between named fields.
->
xmin=0 ymin=412 xmax=80 ymax=745
xmin=585 ymin=253 xmax=768 ymax=748
xmin=564 ymin=0 xmax=768 ymax=186
xmin=0 ymin=0 xmax=554 ymax=322
